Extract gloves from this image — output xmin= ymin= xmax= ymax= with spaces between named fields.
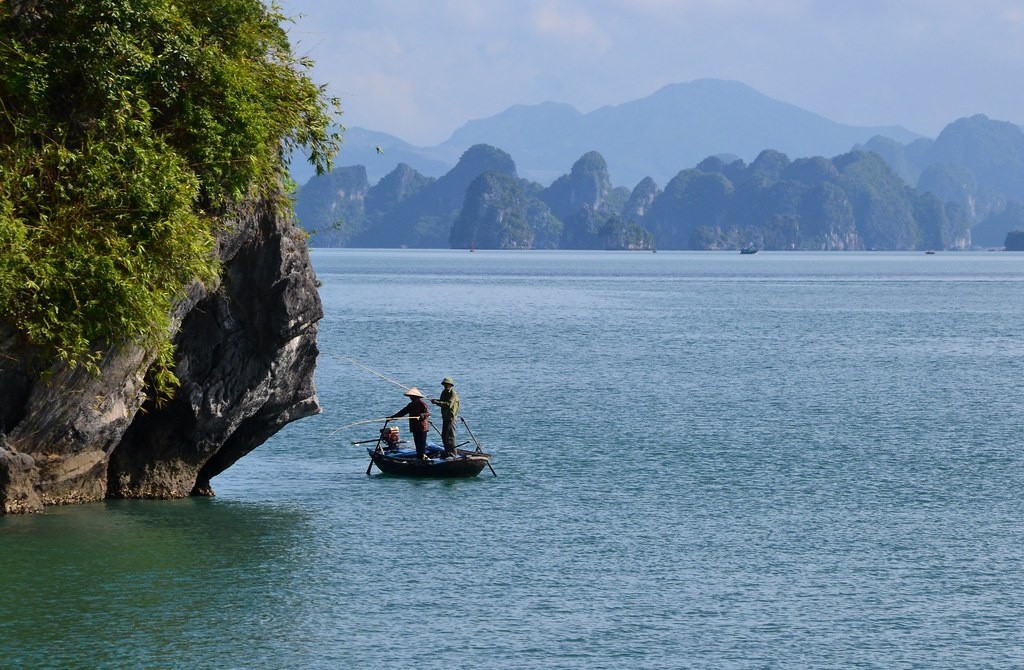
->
xmin=430 ymin=399 xmax=434 ymax=402
xmin=386 ymin=417 xmax=391 ymax=422
xmin=432 ymin=400 xmax=437 ymax=404
xmin=420 ymin=414 xmax=424 ymax=419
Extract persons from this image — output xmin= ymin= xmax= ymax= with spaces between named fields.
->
xmin=386 ymin=386 xmax=431 ymax=460
xmin=430 ymin=377 xmax=460 ymax=458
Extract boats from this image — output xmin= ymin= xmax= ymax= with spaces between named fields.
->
xmin=739 ymin=247 xmax=759 ymax=255
xmin=365 ymin=426 xmax=490 ymax=479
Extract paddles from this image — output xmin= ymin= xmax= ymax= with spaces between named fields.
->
xmin=365 ymin=416 xmax=498 ymax=476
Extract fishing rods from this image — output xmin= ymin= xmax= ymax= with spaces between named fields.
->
xmin=318 ymin=416 xmax=420 ymax=445
xmin=348 ymin=359 xmax=432 ymax=401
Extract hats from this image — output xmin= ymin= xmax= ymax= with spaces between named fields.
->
xmin=441 ymin=378 xmax=454 ymax=386
xmin=403 ymin=387 xmax=426 ymax=398
